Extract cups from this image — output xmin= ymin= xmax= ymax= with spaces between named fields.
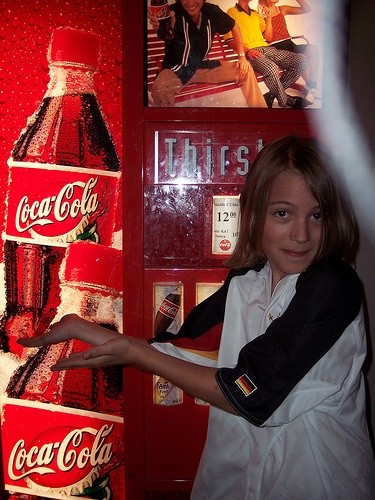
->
xmin=257 ymin=4 xmax=268 ymax=18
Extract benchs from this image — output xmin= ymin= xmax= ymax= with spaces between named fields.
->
xmin=147 ymin=24 xmax=319 ymax=108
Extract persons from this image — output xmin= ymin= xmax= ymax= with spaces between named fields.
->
xmin=147 ymin=0 xmax=267 ymax=108
xmin=224 ymin=0 xmax=308 ymax=108
xmin=15 ymin=135 xmax=375 ymax=500
xmin=258 ymin=0 xmax=322 ymax=103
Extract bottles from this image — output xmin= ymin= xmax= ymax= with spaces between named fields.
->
xmin=155 ymin=377 xmax=175 ymax=403
xmin=151 ymin=0 xmax=172 ymax=40
xmin=0 ymin=239 xmax=122 ymax=500
xmin=155 ymin=286 xmax=181 ymax=336
xmin=3 ymin=27 xmax=121 ymax=360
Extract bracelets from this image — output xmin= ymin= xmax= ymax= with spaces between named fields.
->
xmin=238 ymin=53 xmax=246 ymax=58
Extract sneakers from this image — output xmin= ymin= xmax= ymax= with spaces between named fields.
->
xmin=287 ymin=95 xmax=312 ymax=107
xmin=303 ymin=81 xmax=323 ymax=99
xmin=264 ymin=92 xmax=275 ymax=107
